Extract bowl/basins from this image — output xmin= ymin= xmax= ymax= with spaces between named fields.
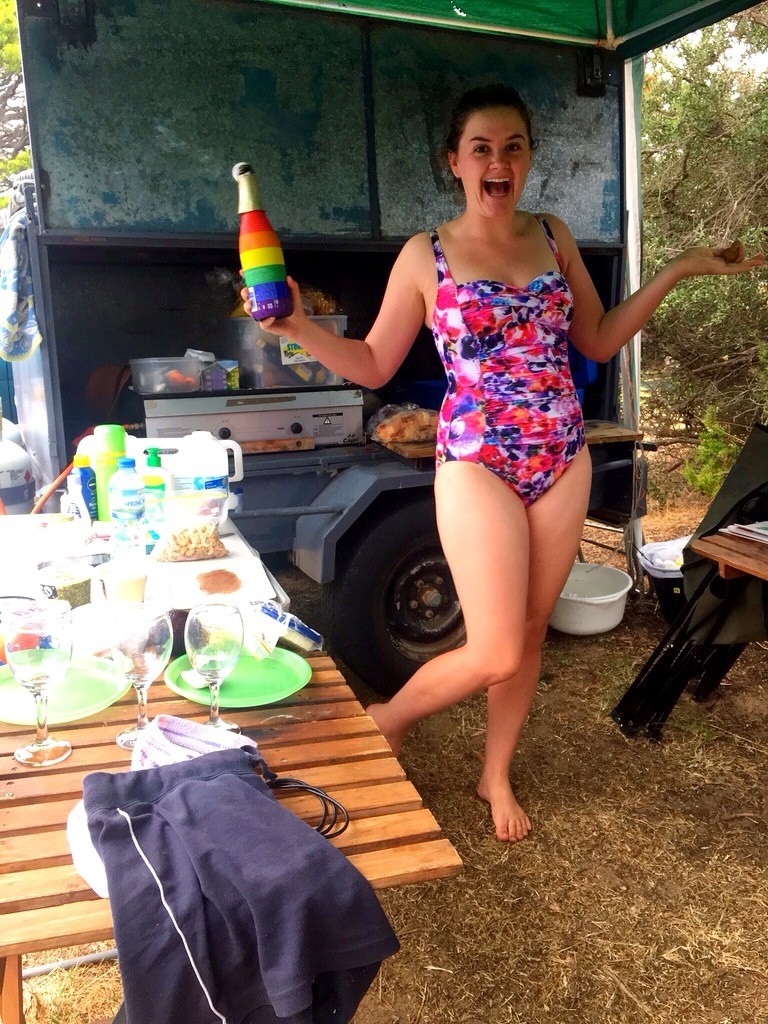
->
xmin=128 ymin=357 xmax=200 ymax=394
xmin=547 ymin=562 xmax=633 ymax=636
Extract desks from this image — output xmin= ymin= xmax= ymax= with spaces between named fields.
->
xmin=0 ymin=518 xmax=464 ymax=1024
xmin=607 ymin=521 xmax=768 ymax=734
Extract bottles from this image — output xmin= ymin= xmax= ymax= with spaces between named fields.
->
xmin=70 ymin=454 xmax=98 ymax=521
xmin=237 ymin=163 xmax=294 ymax=322
xmin=93 ymin=425 xmax=126 ymax=521
xmin=256 ymin=338 xmax=316 ymax=385
xmin=107 ymin=457 xmax=146 ymax=560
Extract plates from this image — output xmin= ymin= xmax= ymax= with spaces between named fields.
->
xmin=0 ymin=657 xmax=133 ymax=726
xmin=142 ymin=555 xmax=278 ymax=607
xmin=163 ymin=647 xmax=312 ymax=708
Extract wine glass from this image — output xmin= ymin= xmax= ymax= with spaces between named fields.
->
xmin=183 ymin=602 xmax=244 ymax=735
xmin=4 ymin=600 xmax=74 ymax=767
xmin=110 ymin=608 xmax=174 ymax=751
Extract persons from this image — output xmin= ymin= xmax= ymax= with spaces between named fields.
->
xmin=239 ymin=84 xmax=764 ymax=843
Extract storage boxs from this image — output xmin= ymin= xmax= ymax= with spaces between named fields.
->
xmin=227 ymin=314 xmax=349 ymax=387
